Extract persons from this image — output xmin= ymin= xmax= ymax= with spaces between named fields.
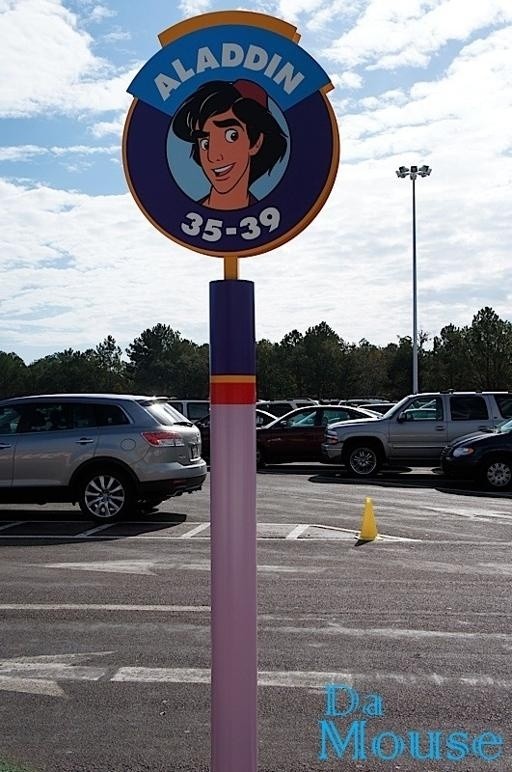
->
xmin=172 ymin=80 xmax=288 ymax=212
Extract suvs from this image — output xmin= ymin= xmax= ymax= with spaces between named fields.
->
xmin=0 ymin=393 xmax=207 ymax=523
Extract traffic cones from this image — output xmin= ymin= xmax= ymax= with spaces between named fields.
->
xmin=355 ymin=497 xmax=382 ymax=542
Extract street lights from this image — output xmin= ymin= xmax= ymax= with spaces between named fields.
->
xmin=395 ymin=164 xmax=433 ymax=392
